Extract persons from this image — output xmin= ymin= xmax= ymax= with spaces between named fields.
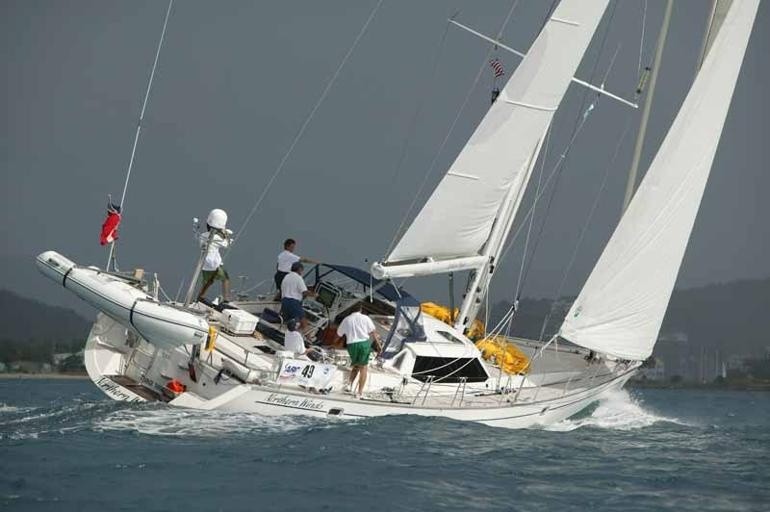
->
xmin=280 ymin=262 xmax=320 ymax=339
xmin=331 ymin=302 xmax=383 ymax=398
xmin=283 ymin=318 xmax=313 ymax=361
xmin=198 ymin=223 xmax=233 ymax=303
xmin=273 ymin=239 xmax=320 ymax=303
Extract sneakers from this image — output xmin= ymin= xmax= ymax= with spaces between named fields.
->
xmin=343 ymin=382 xmax=352 ymax=392
xmin=360 ymin=395 xmax=372 ymax=400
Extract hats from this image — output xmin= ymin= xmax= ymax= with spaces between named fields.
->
xmin=287 ymin=314 xmax=300 ymax=331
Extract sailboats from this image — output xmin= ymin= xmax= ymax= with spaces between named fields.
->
xmin=36 ymin=1 xmax=761 ymax=427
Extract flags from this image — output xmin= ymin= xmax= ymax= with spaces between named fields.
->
xmin=488 ymin=57 xmax=505 ymax=79
xmin=99 ymin=194 xmax=121 ymax=245
